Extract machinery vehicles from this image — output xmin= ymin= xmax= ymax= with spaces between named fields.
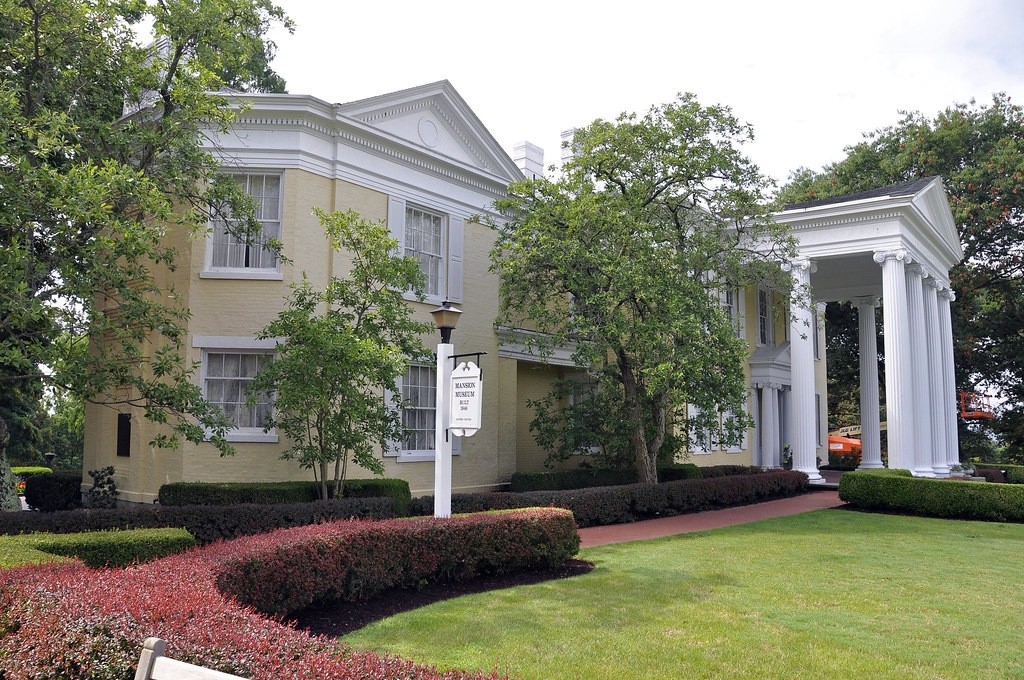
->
xmin=826 ymin=391 xmax=993 ymax=460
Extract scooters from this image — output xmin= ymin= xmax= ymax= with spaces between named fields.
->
xmin=781 ymin=444 xmax=822 ymax=471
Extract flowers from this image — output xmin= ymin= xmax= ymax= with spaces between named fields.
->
xmin=782 ymin=443 xmax=794 ymax=463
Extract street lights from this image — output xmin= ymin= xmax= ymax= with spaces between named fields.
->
xmin=429 ymin=296 xmax=488 ymax=519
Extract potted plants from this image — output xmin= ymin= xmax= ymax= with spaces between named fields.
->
xmin=962 ymin=462 xmax=976 ymax=478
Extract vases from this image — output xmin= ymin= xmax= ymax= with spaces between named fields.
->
xmin=783 ymin=462 xmax=792 ymax=471
